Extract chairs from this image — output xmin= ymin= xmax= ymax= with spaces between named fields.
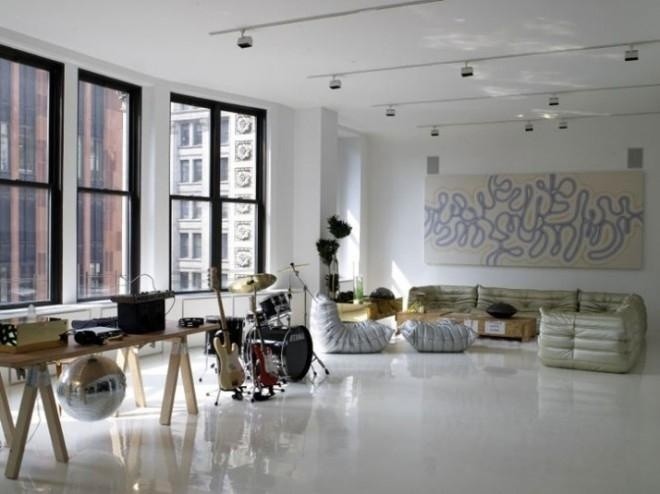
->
xmin=310 ymin=292 xmax=395 ymax=353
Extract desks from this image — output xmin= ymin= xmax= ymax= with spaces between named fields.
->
xmin=395 ymin=312 xmax=537 ymax=344
xmin=0 ymin=319 xmax=220 ymax=480
xmin=336 ymin=296 xmax=402 ymax=322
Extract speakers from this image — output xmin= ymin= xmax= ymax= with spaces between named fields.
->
xmin=204 ymin=311 xmax=243 ymax=355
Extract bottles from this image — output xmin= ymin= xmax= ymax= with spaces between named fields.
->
xmin=26 ymin=303 xmax=38 ymax=325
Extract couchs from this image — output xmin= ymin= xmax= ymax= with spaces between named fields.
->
xmin=399 ymin=284 xmax=646 ymax=374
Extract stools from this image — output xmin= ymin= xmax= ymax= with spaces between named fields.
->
xmin=399 ymin=318 xmax=478 ymax=353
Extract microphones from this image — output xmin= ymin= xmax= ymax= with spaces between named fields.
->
xmin=289 ymin=261 xmax=299 ymax=274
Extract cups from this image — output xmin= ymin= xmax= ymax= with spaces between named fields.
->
xmin=353 ymin=275 xmax=363 ymax=304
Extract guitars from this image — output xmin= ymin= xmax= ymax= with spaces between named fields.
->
xmin=207 ymin=267 xmax=245 ymax=391
xmin=250 ymin=295 xmax=279 ymax=388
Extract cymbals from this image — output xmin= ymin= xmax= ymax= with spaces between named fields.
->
xmin=229 ymin=272 xmax=277 ymax=294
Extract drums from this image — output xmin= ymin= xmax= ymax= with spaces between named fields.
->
xmin=260 ymin=292 xmax=291 ymax=325
xmin=242 ymin=323 xmax=314 ymax=383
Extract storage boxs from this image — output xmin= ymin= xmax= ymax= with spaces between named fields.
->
xmin=0 ymin=317 xmax=69 ymax=354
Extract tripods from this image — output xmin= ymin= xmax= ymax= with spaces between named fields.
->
xmin=247 ymin=291 xmax=287 ymax=402
xmin=289 ymin=265 xmax=330 ymax=378
xmin=210 ymin=298 xmax=249 ymax=404
